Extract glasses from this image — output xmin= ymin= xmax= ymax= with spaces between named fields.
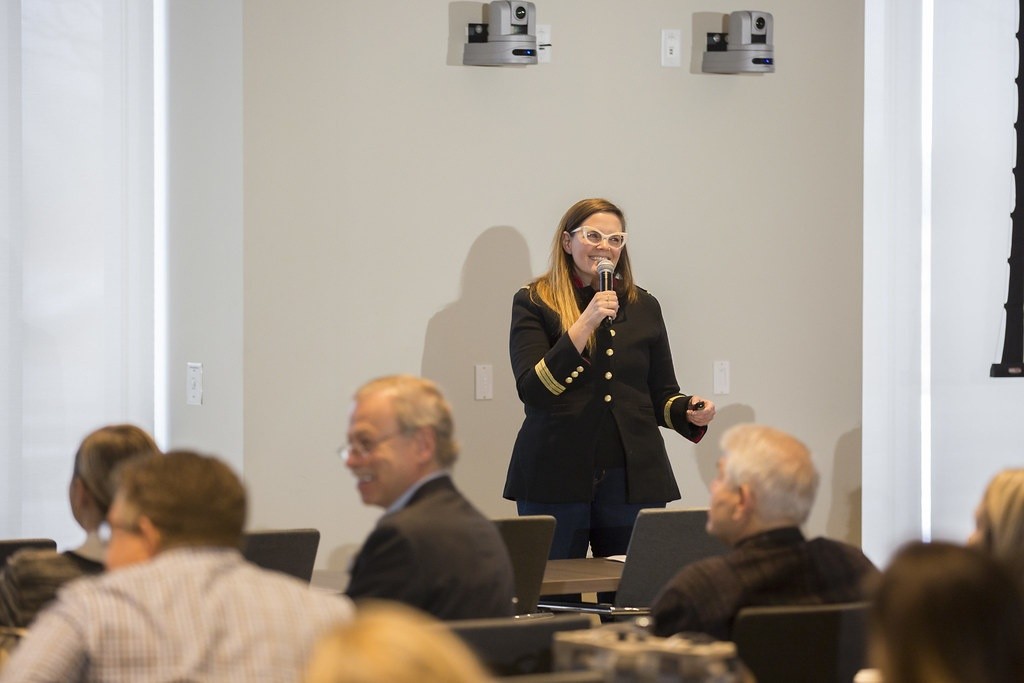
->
xmin=569 ymin=226 xmax=629 ymax=250
xmin=337 ymin=429 xmax=400 ymax=461
xmin=96 ymin=518 xmax=142 ymax=547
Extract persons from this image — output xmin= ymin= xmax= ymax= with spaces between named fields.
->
xmin=343 ymin=374 xmax=515 ymax=621
xmin=304 ymin=600 xmax=491 ymax=683
xmin=963 ymin=465 xmax=1024 ymax=571
xmin=503 ymin=198 xmax=716 ymax=625
xmin=647 ymin=422 xmax=885 ymax=644
xmin=851 ymin=539 xmax=1024 ymax=683
xmin=0 ymin=451 xmax=356 ymax=683
xmin=59 ymin=424 xmax=165 ymax=576
xmin=0 ymin=548 xmax=87 ymax=671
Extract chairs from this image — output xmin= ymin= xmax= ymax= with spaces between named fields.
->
xmin=0 ymin=509 xmax=874 ymax=683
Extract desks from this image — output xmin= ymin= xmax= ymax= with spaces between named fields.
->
xmin=304 ymin=555 xmax=626 ymax=592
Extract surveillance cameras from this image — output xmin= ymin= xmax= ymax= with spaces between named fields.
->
xmin=702 ymin=11 xmax=775 ymax=74
xmin=462 ymin=0 xmax=539 ymax=66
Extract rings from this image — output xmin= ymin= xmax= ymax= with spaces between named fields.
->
xmin=607 ymin=294 xmax=610 ymax=301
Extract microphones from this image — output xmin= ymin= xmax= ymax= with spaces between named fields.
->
xmin=596 ymin=260 xmax=615 ymax=327
